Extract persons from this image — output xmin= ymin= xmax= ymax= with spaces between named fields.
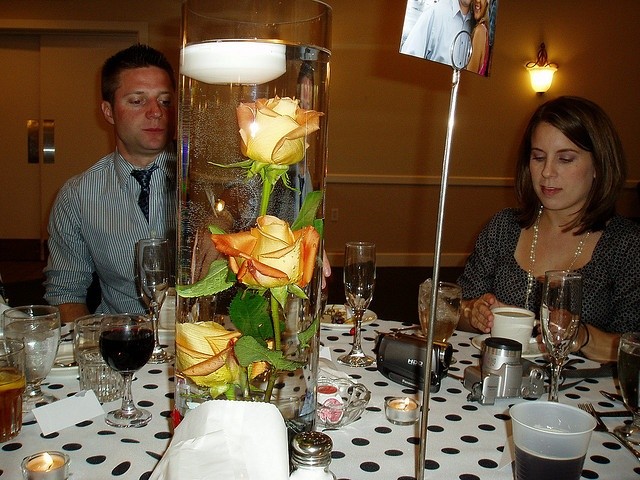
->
xmin=467 ymin=0 xmax=493 ymax=76
xmin=42 ymin=44 xmax=226 ymax=331
xmin=455 ymin=94 xmax=640 ymax=363
xmin=398 ymin=0 xmax=473 ymax=69
xmin=274 ymin=57 xmax=317 ymax=226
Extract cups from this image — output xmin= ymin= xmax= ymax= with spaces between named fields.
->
xmin=489 ymin=307 xmax=541 ymax=351
xmin=75 ymin=316 xmax=126 ymax=401
xmin=0 ymin=339 xmax=25 ymax=443
xmin=508 ymin=400 xmax=597 ymax=480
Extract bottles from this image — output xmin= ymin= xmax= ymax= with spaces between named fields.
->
xmin=291 ymin=432 xmax=337 ymax=480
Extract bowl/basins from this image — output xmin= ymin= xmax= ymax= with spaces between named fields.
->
xmin=316 ymin=375 xmax=371 ymax=428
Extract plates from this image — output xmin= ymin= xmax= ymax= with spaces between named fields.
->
xmin=320 ymin=304 xmax=376 ymax=330
xmin=471 ymin=333 xmax=549 ymax=358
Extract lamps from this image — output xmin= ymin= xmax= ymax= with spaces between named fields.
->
xmin=525 ymin=43 xmax=559 ymax=94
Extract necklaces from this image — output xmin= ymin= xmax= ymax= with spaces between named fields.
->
xmin=525 ymin=202 xmax=590 ymax=317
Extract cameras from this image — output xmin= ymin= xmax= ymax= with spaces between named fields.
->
xmin=464 ymin=337 xmax=544 ymax=406
xmin=374 ymin=330 xmax=453 ymax=394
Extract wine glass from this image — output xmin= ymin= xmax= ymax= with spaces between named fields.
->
xmin=135 ymin=239 xmax=175 ymax=363
xmin=100 ymin=314 xmax=156 ymax=429
xmin=337 ymin=242 xmax=378 ymax=367
xmin=419 ymin=282 xmax=464 ymax=364
xmin=540 ymin=270 xmax=582 ymax=402
xmin=614 ymin=332 xmax=639 ymax=443
xmin=3 ymin=305 xmax=61 ymax=412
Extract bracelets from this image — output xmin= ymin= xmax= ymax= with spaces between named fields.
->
xmin=577 ymin=318 xmax=591 ymax=355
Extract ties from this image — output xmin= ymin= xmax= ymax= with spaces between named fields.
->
xmin=130 ymin=163 xmax=159 ymax=224
